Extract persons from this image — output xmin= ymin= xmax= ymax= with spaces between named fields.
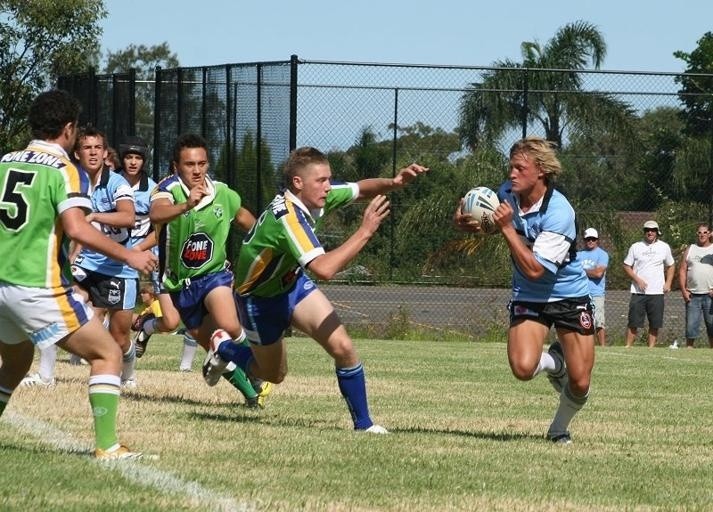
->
xmin=573 ymin=224 xmax=607 ymax=347
xmin=0 ymin=90 xmax=160 ymax=460
xmin=19 ymin=125 xmax=269 ymax=408
xmin=622 ymin=220 xmax=675 ymax=349
xmin=201 ymin=146 xmax=430 ymax=435
xmin=678 ymin=225 xmax=712 ymax=348
xmin=449 ymin=134 xmax=593 ymax=448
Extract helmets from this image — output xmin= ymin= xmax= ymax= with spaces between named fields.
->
xmin=114 ymin=135 xmax=153 ymax=171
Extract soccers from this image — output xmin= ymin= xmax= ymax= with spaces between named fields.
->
xmin=462 ymin=186 xmax=499 ymax=234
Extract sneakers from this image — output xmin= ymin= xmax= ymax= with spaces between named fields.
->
xmin=69 ymin=354 xmax=89 ymax=365
xmin=245 ymin=373 xmax=271 ymax=397
xmin=245 ymin=394 xmax=265 ymax=411
xmin=547 ymin=342 xmax=569 ymax=393
xmin=120 ymin=370 xmax=137 ymax=388
xmin=202 ymin=328 xmax=232 ymax=387
xmin=545 ymin=425 xmax=572 ymax=446
xmin=354 ymin=424 xmax=392 ymax=435
xmin=94 ymin=443 xmax=144 ymax=463
xmin=132 ymin=312 xmax=155 ymax=359
xmin=19 ymin=372 xmax=55 ymax=390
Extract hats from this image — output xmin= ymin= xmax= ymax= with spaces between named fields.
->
xmin=639 ymin=221 xmax=662 ymax=235
xmin=582 ymin=228 xmax=598 ymax=239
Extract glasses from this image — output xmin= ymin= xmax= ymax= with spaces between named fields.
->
xmin=695 ymin=230 xmax=710 ymax=236
